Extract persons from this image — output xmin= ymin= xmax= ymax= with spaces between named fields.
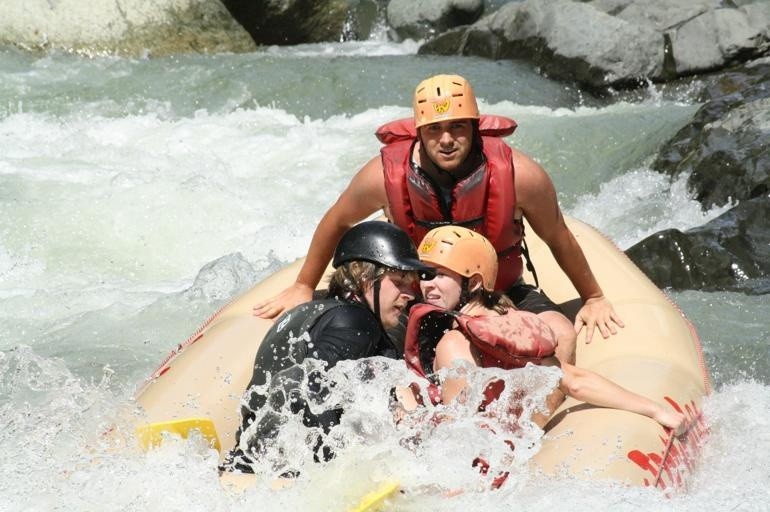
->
xmin=405 ymin=226 xmax=688 ymax=451
xmin=253 ymin=73 xmax=627 ymax=345
xmin=226 ymin=220 xmax=429 ymax=480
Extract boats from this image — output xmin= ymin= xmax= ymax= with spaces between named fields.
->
xmin=75 ymin=207 xmax=713 ymax=501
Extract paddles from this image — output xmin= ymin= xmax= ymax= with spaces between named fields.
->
xmin=348 ymin=482 xmax=399 ymax=510
xmin=137 ymin=420 xmax=221 ymax=459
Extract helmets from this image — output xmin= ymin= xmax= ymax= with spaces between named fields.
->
xmin=411 ymin=74 xmax=480 ymax=129
xmin=416 ymin=224 xmax=499 ymax=292
xmin=331 ymin=221 xmax=436 ymax=281
